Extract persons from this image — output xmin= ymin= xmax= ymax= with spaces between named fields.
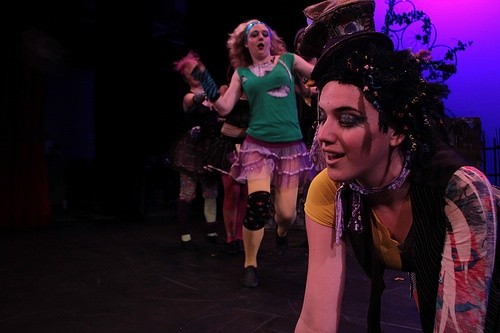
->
xmin=294 ymin=32 xmax=500 ymax=333
xmin=174 ymin=19 xmax=327 ymax=287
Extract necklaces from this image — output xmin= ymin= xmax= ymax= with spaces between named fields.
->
xmin=190 ymin=89 xmax=197 ymax=94
xmin=253 ymin=56 xmax=275 ymax=65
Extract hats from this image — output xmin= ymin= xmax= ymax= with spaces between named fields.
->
xmin=297 ymin=0 xmax=394 ymax=80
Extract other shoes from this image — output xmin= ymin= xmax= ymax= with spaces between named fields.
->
xmin=277 ymin=232 xmax=288 ymax=251
xmin=207 ymin=234 xmax=227 ymax=246
xmin=181 ymin=239 xmax=201 ymax=251
xmin=240 ymin=265 xmax=259 ymax=287
